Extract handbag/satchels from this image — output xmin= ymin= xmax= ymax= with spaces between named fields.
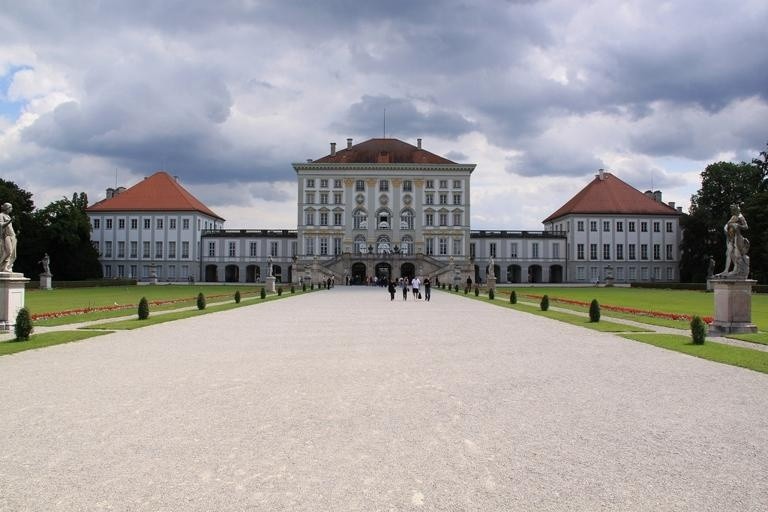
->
xmin=417 ymin=292 xmax=422 ymax=299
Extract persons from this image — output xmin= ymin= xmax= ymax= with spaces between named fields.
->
xmin=453 ymin=272 xmax=461 ymax=286
xmin=323 ymin=274 xmax=336 ymax=290
xmin=467 ymin=275 xmax=472 ymax=293
xmin=38 ymin=253 xmax=51 ymax=274
xmin=436 ymin=276 xmax=439 ymax=286
xmin=256 ymin=273 xmax=260 ymax=284
xmin=345 ymin=273 xmax=431 ymax=301
xmin=1 ymin=203 xmax=17 ymax=272
xmin=716 ymin=202 xmax=749 ymax=278
